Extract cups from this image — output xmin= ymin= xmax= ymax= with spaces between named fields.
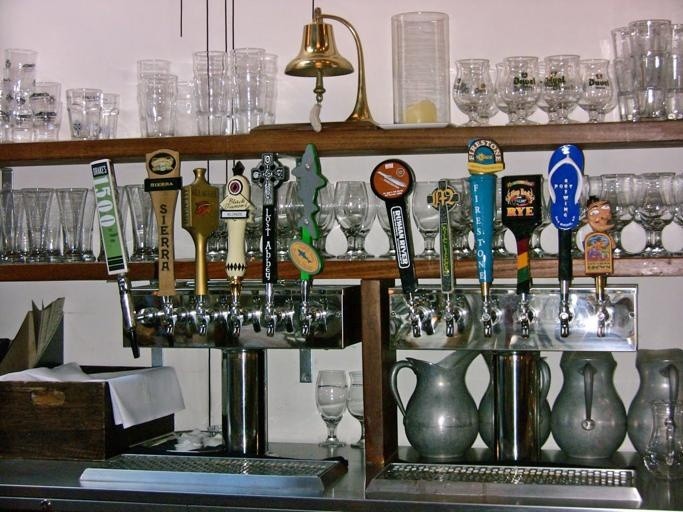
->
xmin=4 ymin=44 xmax=280 ymax=142
xmin=3 ymin=171 xmax=682 ymax=263
xmin=451 ymin=18 xmax=682 ymax=123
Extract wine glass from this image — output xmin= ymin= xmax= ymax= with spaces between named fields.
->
xmin=314 ymin=369 xmax=348 ymax=448
xmin=346 ymin=370 xmax=368 ymax=450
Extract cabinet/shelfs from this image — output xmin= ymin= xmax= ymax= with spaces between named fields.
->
xmin=0 ymin=119 xmax=680 ymax=506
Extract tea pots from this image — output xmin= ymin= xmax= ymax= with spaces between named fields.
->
xmin=388 ymin=348 xmax=683 ymax=484
